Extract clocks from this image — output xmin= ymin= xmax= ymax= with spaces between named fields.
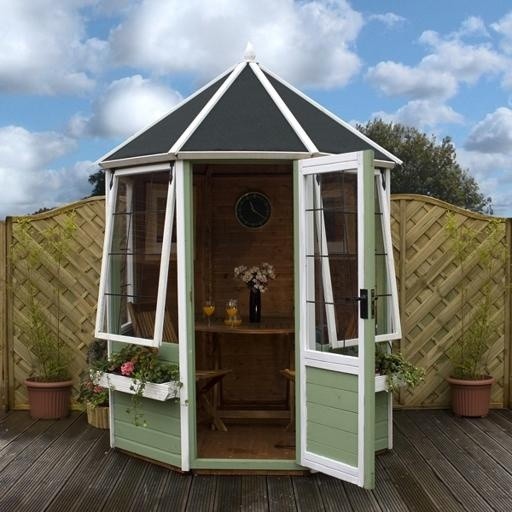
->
xmin=234 ymin=191 xmax=274 ymax=231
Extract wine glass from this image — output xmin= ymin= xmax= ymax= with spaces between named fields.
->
xmin=202 ymin=301 xmax=217 ymax=329
xmin=225 ymin=299 xmax=239 ymax=330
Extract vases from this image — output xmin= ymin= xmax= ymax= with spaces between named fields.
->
xmin=89 ymin=369 xmax=179 ymax=403
xmin=86 ymin=400 xmax=110 ymax=429
xmin=249 ymin=290 xmax=262 ymax=323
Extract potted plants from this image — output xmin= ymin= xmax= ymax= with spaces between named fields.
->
xmin=19 ymin=208 xmax=75 ymax=420
xmin=445 ymin=210 xmax=509 ymax=418
xmin=375 ymin=352 xmax=426 ymax=393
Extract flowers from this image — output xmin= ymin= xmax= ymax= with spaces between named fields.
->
xmin=89 ymin=344 xmax=192 ymax=432
xmin=75 ymin=340 xmax=109 ymax=407
xmin=233 ymin=263 xmax=277 ymax=294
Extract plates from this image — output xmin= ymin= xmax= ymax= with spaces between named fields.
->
xmin=225 ymin=320 xmax=242 ymax=325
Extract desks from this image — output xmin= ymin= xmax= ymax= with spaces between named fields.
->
xmin=195 ymin=316 xmax=295 ymax=431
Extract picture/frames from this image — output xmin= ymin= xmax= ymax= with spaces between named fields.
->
xmin=144 ymin=180 xmax=200 ymax=262
xmin=321 ymin=181 xmax=358 ymax=261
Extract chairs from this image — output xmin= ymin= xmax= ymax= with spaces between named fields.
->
xmin=274 ymin=313 xmax=359 ymax=448
xmin=126 ymin=300 xmax=229 ymax=433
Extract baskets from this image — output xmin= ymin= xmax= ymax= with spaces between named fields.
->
xmin=86 ymin=397 xmax=110 ymax=430
